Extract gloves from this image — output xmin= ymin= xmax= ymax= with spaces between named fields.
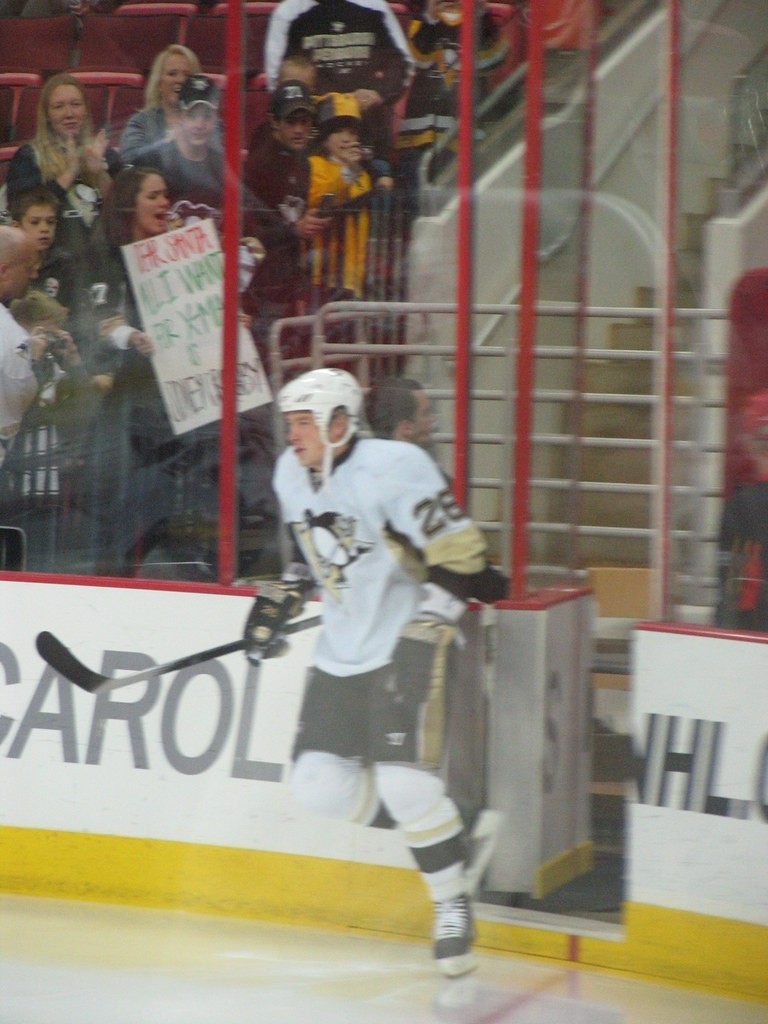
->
xmin=393 ymin=625 xmax=440 ymax=706
xmin=244 ymin=583 xmax=304 ymax=667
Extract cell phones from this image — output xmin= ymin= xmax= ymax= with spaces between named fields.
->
xmin=317 ymin=193 xmax=334 ymax=218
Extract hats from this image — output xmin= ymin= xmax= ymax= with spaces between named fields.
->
xmin=180 ymin=74 xmax=219 ymax=110
xmin=314 ymin=92 xmax=362 ymax=124
xmin=272 ymin=80 xmax=315 ymax=119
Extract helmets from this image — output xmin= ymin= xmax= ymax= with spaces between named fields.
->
xmin=278 ymin=368 xmax=362 ymax=447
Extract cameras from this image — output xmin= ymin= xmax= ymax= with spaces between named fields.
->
xmin=40 ymin=330 xmax=60 ymax=353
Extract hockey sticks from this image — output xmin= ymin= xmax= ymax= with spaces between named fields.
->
xmin=36 ymin=614 xmax=325 ymax=696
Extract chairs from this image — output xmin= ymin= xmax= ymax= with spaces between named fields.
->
xmin=0 ymin=0 xmax=596 ymax=202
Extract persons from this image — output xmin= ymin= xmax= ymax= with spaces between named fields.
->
xmin=715 ymin=387 xmax=768 ymax=637
xmin=244 ymin=369 xmax=510 ymax=977
xmin=0 ymin=0 xmax=512 ymax=575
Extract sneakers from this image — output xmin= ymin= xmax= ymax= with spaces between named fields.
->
xmin=454 ymin=799 xmax=504 ymax=894
xmin=434 ymin=892 xmax=479 ymax=977
